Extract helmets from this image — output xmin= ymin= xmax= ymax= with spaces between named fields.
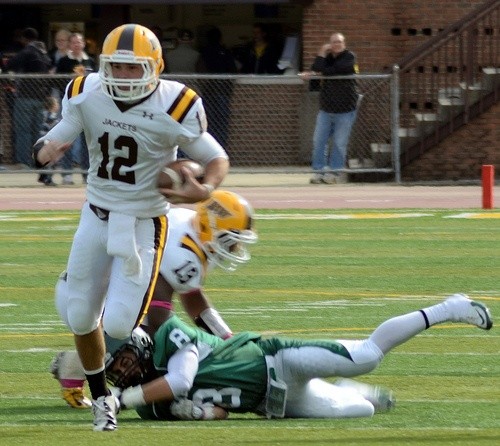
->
xmin=103 ymin=325 xmax=155 ymax=389
xmin=193 ymin=190 xmax=258 ymax=271
xmin=97 ymin=23 xmax=164 ymax=102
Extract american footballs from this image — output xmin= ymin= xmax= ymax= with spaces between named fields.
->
xmin=156 ymin=157 xmax=205 ymax=206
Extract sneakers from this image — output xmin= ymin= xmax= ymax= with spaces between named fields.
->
xmin=335 ymin=377 xmax=393 ymax=415
xmin=446 ymin=292 xmax=493 ymax=331
xmin=50 ymin=351 xmax=92 ymax=408
xmin=91 ymin=388 xmax=121 ymax=432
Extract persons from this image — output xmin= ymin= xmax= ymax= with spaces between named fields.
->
xmin=31 ymin=24 xmax=230 ymax=430
xmin=2 ymin=21 xmax=272 ymax=186
xmin=50 ymin=190 xmax=257 ymax=408
xmin=104 ymin=292 xmax=493 ymax=421
xmin=300 ymin=31 xmax=359 ymax=183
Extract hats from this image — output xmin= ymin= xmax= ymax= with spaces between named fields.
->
xmin=18 ymin=27 xmax=38 ymax=40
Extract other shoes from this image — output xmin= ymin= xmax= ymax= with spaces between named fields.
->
xmin=62 ymin=173 xmax=74 ymax=186
xmin=38 ymin=175 xmax=57 ymax=186
xmin=310 ymin=173 xmax=325 ymax=184
xmin=323 ymin=173 xmax=344 ymax=184
